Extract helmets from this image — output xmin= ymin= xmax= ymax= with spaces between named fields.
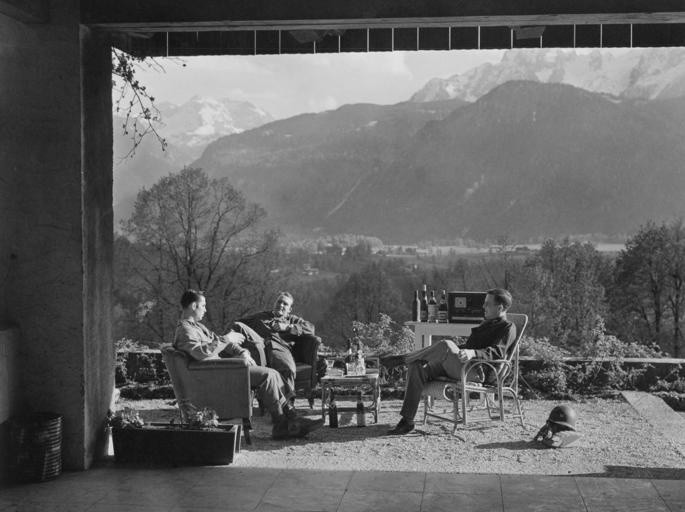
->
xmin=547 ymin=405 xmax=578 ymax=430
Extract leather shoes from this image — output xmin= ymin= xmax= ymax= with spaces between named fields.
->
xmin=391 ymin=420 xmax=414 ymax=436
xmin=381 ymin=353 xmax=403 ymax=367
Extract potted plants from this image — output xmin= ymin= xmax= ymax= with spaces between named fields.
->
xmin=106 ymin=399 xmax=242 ymax=466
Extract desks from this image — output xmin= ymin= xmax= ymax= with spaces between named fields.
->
xmin=405 ymin=319 xmax=482 ymax=353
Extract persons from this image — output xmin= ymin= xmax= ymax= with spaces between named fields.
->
xmin=236 ymin=292 xmax=314 ymax=410
xmin=379 ymin=288 xmax=516 ymax=434
xmin=173 ymin=289 xmax=324 ymax=440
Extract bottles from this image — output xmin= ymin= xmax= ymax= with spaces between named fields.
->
xmin=328 ymin=388 xmax=339 ymax=429
xmin=356 ymin=390 xmax=366 ymax=427
xmin=344 ymin=336 xmax=365 ymax=377
xmin=412 ymin=284 xmax=448 ymax=324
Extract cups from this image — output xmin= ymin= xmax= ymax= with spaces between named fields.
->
xmin=325 ymin=359 xmax=335 ymax=368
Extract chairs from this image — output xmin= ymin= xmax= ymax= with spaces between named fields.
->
xmin=422 ymin=309 xmax=528 ymax=435
xmin=163 ymin=332 xmax=322 ymax=446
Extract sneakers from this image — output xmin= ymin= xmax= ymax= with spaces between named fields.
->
xmin=275 ymin=407 xmax=323 ymax=440
